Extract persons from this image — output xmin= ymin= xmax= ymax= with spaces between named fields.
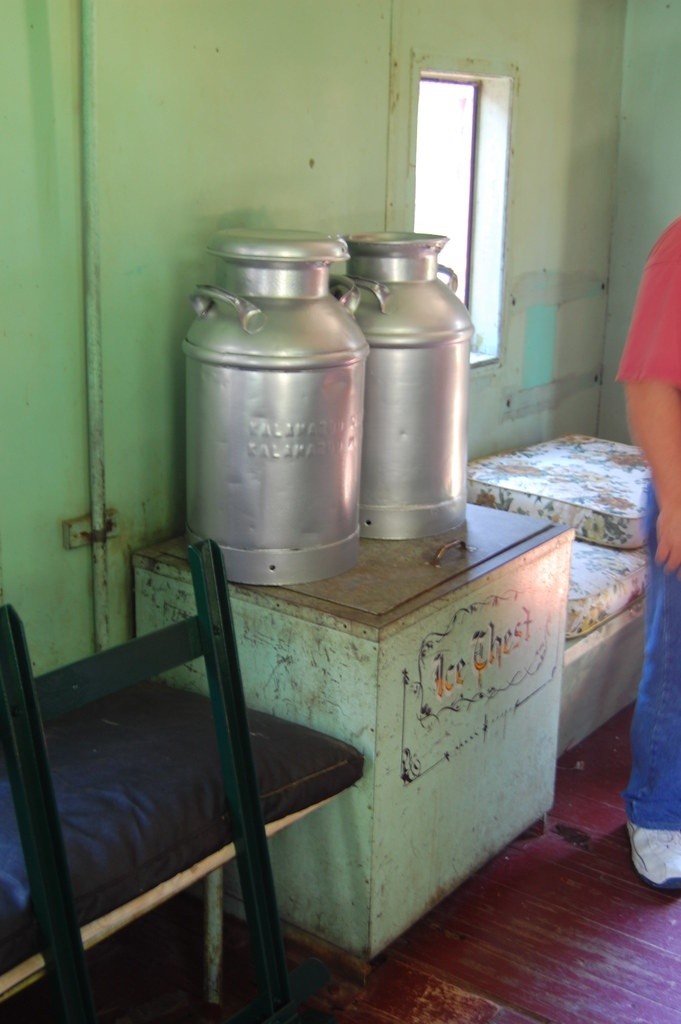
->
xmin=614 ymin=218 xmax=681 ymax=892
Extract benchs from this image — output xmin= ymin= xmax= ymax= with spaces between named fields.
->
xmin=472 ymin=429 xmax=651 ymax=757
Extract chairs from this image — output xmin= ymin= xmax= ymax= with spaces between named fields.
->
xmin=0 ymin=539 xmax=368 ymax=1024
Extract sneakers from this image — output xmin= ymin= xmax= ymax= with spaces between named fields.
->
xmin=626 ymin=818 xmax=681 ymax=891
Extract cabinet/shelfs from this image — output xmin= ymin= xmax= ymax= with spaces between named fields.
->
xmin=133 ymin=502 xmax=577 ymax=981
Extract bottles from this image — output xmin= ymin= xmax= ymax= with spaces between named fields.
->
xmin=184 ymin=232 xmax=474 ymax=584
